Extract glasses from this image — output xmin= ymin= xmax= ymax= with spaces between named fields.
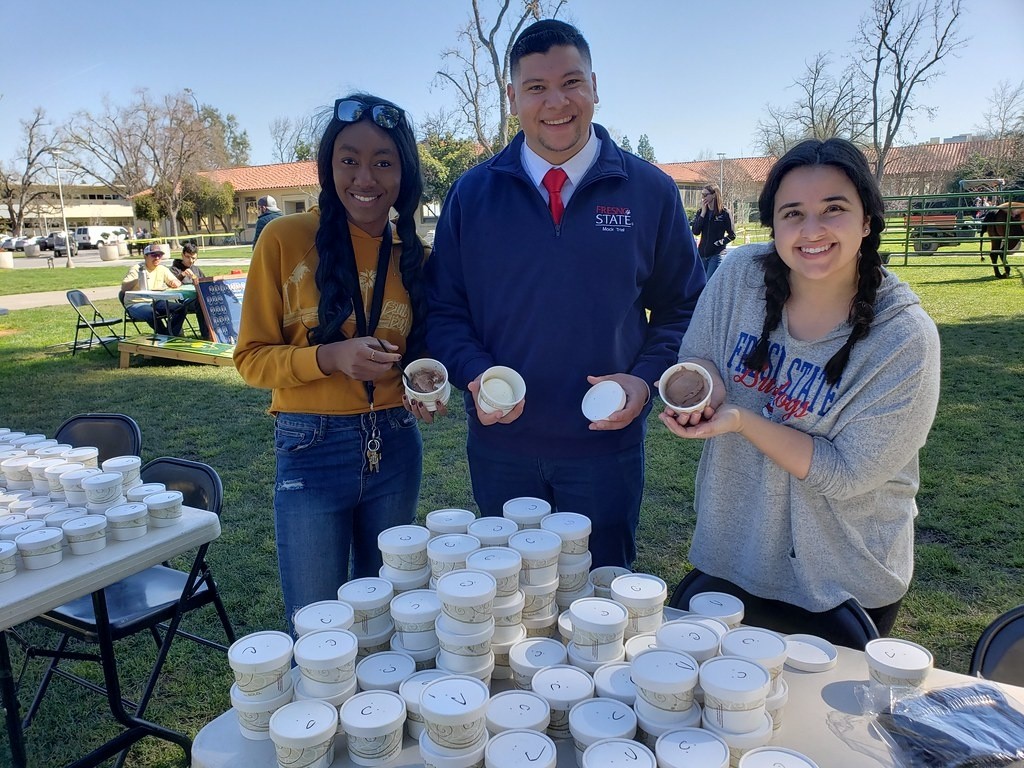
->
xmin=702 ymin=192 xmax=713 ymax=197
xmin=334 ymin=97 xmax=409 ymax=132
xmin=149 ymin=253 xmax=162 ymax=259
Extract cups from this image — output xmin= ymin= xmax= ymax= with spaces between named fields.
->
xmin=226 ymin=494 xmax=820 ymax=768
xmin=477 ymin=365 xmax=526 ymax=418
xmin=402 ymin=357 xmax=451 ymax=412
xmin=0 ymin=425 xmax=184 ymax=585
xmin=865 ymin=637 xmax=933 ymax=706
xmin=658 ymin=361 xmax=714 ymax=416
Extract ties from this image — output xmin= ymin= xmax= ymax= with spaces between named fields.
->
xmin=541 ymin=168 xmax=568 ymax=225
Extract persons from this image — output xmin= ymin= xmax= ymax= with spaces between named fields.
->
xmin=252 ymin=195 xmax=284 ymax=249
xmin=125 ymin=226 xmax=151 ymax=255
xmin=122 ymin=245 xmax=187 ymax=337
xmin=692 ymin=184 xmax=736 ymax=279
xmin=233 ymin=96 xmax=447 ymax=641
xmin=171 ymin=244 xmax=210 ymax=339
xmin=653 ymin=137 xmax=940 ymax=636
xmin=423 ymin=19 xmax=708 ymax=571
xmin=972 ymin=198 xmax=995 ymax=228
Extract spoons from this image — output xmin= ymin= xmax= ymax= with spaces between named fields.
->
xmin=375 ymin=336 xmax=439 ymax=393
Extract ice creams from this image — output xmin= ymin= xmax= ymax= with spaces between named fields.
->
xmin=483 ymin=378 xmax=516 ymax=404
xmin=408 ymin=368 xmax=445 ymax=394
xmin=665 ymin=366 xmax=710 ymax=408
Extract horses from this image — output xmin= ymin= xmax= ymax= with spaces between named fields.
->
xmin=980 ymin=201 xmax=1024 ymax=277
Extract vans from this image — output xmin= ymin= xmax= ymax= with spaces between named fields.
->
xmin=75 ymin=226 xmax=129 ymax=250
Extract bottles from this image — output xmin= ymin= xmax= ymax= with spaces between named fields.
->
xmin=138 ymin=265 xmax=148 ymax=291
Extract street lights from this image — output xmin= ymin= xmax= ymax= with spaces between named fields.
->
xmin=717 ymin=152 xmax=726 ymax=194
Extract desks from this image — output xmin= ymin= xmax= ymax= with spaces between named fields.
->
xmin=124 ymin=284 xmax=199 ymax=337
xmin=0 ymin=501 xmax=223 ymax=768
xmin=187 ymin=605 xmax=1024 ymax=768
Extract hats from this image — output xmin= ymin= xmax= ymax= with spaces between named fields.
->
xmin=144 ymin=245 xmax=164 ymax=256
xmin=257 ymin=195 xmax=280 ymax=212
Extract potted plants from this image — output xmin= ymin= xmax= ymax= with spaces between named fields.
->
xmin=23 ymin=232 xmax=41 ymax=257
xmin=111 ymin=229 xmax=127 ymax=256
xmin=99 ymin=232 xmax=119 ymax=261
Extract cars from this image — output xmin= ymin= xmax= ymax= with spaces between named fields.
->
xmin=0 ymin=230 xmax=77 ymax=258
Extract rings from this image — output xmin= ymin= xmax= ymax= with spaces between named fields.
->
xmin=370 ymin=350 xmax=377 ymax=361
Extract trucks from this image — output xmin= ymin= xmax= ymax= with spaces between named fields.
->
xmin=903 ymin=179 xmax=1021 ymax=256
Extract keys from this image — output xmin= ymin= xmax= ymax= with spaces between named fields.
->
xmin=367 ymin=438 xmax=382 ymax=473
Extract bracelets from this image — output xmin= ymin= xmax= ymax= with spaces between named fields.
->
xmin=643 ymin=380 xmax=651 ymax=406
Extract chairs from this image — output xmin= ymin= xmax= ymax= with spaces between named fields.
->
xmin=667 ymin=564 xmax=881 ymax=653
xmin=22 ymin=456 xmax=239 ymax=768
xmin=969 ymin=604 xmax=1024 ymax=688
xmin=66 ymin=289 xmax=123 ymax=359
xmin=47 ymin=411 xmax=141 ymax=458
xmin=118 ymin=288 xmax=199 ymax=339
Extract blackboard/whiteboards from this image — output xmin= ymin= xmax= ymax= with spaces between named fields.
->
xmin=192 ymin=273 xmax=248 ymax=346
